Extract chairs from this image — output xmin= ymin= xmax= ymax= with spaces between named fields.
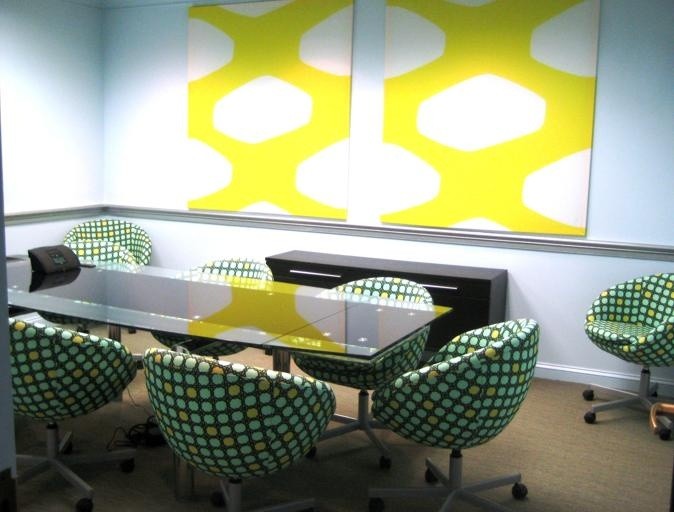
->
xmin=372 ymin=318 xmax=540 ymax=512
xmin=142 ymin=350 xmax=336 ymax=512
xmin=150 ymin=259 xmax=271 ymax=360
xmin=62 ymin=220 xmax=152 ymax=340
xmin=290 ymin=276 xmax=436 ymax=469
xmin=9 ymin=318 xmax=137 ymax=511
xmin=583 ymin=273 xmax=674 ymax=441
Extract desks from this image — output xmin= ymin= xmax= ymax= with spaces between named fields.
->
xmin=6 ymin=258 xmax=451 ymax=402
xmin=265 ymin=250 xmax=510 ymax=366
